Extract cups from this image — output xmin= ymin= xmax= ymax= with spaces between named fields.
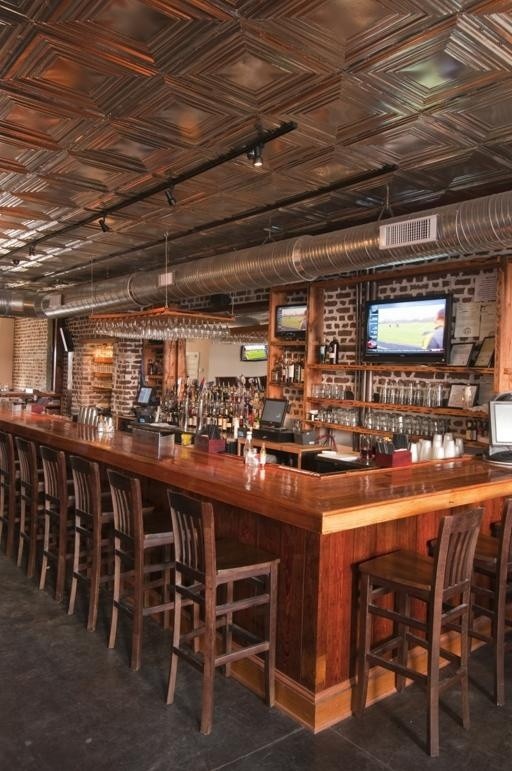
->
xmin=408 ymin=433 xmax=464 ymax=463
xmin=76 ymin=426 xmax=97 ymax=443
xmin=310 ymin=381 xmax=446 ymax=434
xmin=360 ymin=435 xmax=396 ymax=471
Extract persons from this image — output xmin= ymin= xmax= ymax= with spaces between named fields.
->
xmin=428 ymin=309 xmax=445 ymax=350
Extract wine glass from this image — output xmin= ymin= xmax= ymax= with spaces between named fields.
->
xmin=94 ymin=316 xmax=229 ymax=344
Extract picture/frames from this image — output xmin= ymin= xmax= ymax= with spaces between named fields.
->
xmin=444 ymin=342 xmax=475 ymax=366
xmin=473 ymin=336 xmax=495 ymax=368
xmin=447 ymin=383 xmax=480 ymax=409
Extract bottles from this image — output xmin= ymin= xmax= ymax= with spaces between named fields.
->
xmin=244 ymin=431 xmax=266 ymax=463
xmin=465 ymin=421 xmax=488 ymax=441
xmin=162 ymin=375 xmax=263 ymax=433
xmin=272 ymin=350 xmax=305 ymax=385
xmin=76 ymin=405 xmax=98 ymax=426
xmin=319 ymin=334 xmax=339 ymax=365
xmin=244 ymin=463 xmax=268 ymax=490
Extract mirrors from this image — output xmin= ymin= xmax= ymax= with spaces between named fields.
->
xmin=176 ymin=301 xmax=270 ymax=397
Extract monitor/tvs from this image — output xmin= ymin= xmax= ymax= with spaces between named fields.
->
xmin=275 ymin=303 xmax=308 ymax=338
xmin=241 ymin=345 xmax=268 ymax=361
xmin=260 ymin=398 xmax=290 ymax=431
xmin=360 ymin=292 xmax=453 ymax=364
xmin=136 ymin=386 xmax=154 ymax=406
xmin=488 ymin=400 xmax=512 ymax=463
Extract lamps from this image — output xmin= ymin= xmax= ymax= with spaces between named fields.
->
xmin=0 ymin=119 xmax=296 ymax=267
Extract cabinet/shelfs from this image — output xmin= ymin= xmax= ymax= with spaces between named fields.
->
xmin=93 ymin=357 xmax=114 ymax=393
xmin=308 ymin=362 xmax=496 ymax=450
xmin=142 ymin=337 xmax=177 ymax=403
xmin=267 ymin=285 xmax=324 ymax=433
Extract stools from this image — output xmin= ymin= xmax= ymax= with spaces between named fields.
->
xmin=105 ymin=469 xmax=191 ymax=673
xmin=39 ymin=442 xmax=96 ymax=601
xmin=164 ymin=489 xmax=278 ymax=734
xmin=355 ymin=498 xmax=512 ymax=758
xmin=0 ymin=429 xmax=70 ymax=581
xmin=69 ymin=450 xmax=152 ymax=636
